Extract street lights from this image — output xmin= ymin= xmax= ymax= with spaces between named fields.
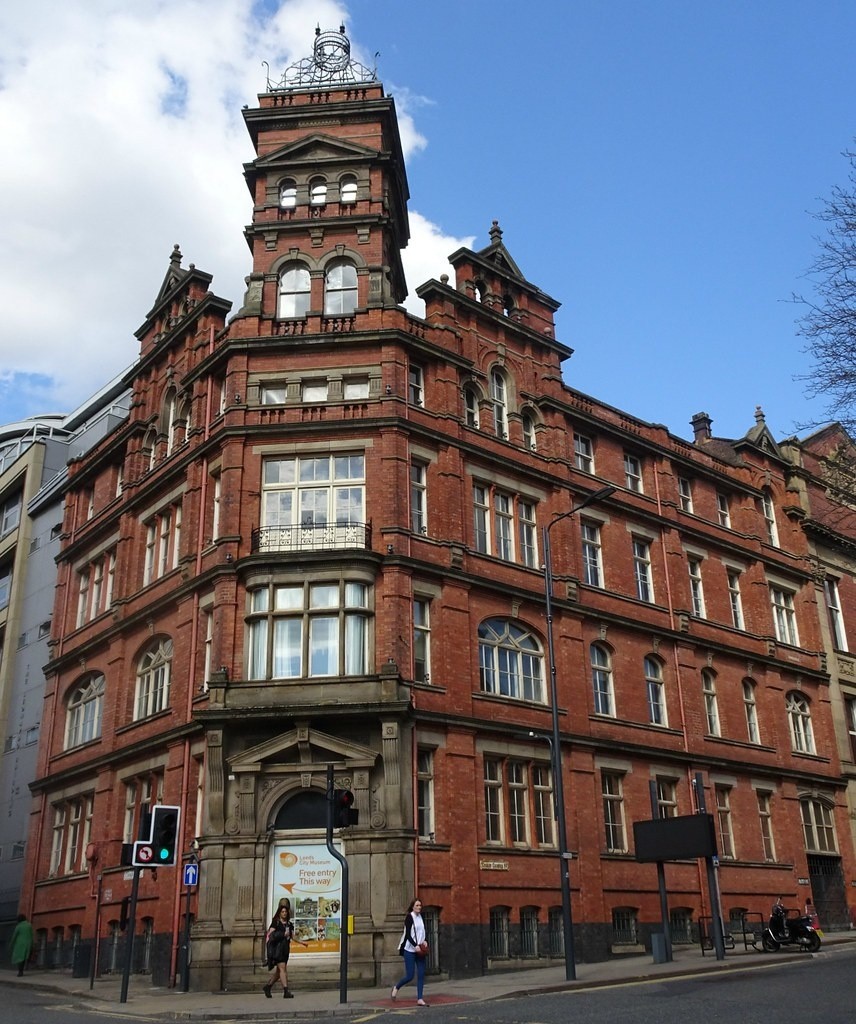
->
xmin=513 ymin=484 xmax=620 ymax=979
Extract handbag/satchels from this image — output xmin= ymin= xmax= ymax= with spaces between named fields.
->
xmin=418 ymin=944 xmax=430 ymax=956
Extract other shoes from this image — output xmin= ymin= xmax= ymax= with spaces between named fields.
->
xmin=391 ymin=986 xmax=396 ymax=1001
xmin=418 ymin=1003 xmax=430 ymax=1007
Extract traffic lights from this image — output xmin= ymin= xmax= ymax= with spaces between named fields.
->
xmin=334 ymin=788 xmax=355 ymax=832
xmin=131 ymin=803 xmax=180 ymax=867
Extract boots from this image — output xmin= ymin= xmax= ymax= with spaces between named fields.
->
xmin=263 ymin=984 xmax=273 ymax=998
xmin=283 ymin=987 xmax=294 ymax=998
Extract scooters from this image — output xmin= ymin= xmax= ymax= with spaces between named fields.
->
xmin=761 ymin=895 xmax=821 ymax=954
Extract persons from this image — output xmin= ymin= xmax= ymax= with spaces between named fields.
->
xmin=804 ymin=897 xmax=816 ymax=916
xmin=262 ymin=904 xmax=308 ymax=999
xmin=8 ymin=913 xmax=34 ymax=977
xmin=391 ymin=897 xmax=432 ymax=1007
xmin=772 ymin=897 xmax=784 ymax=907
